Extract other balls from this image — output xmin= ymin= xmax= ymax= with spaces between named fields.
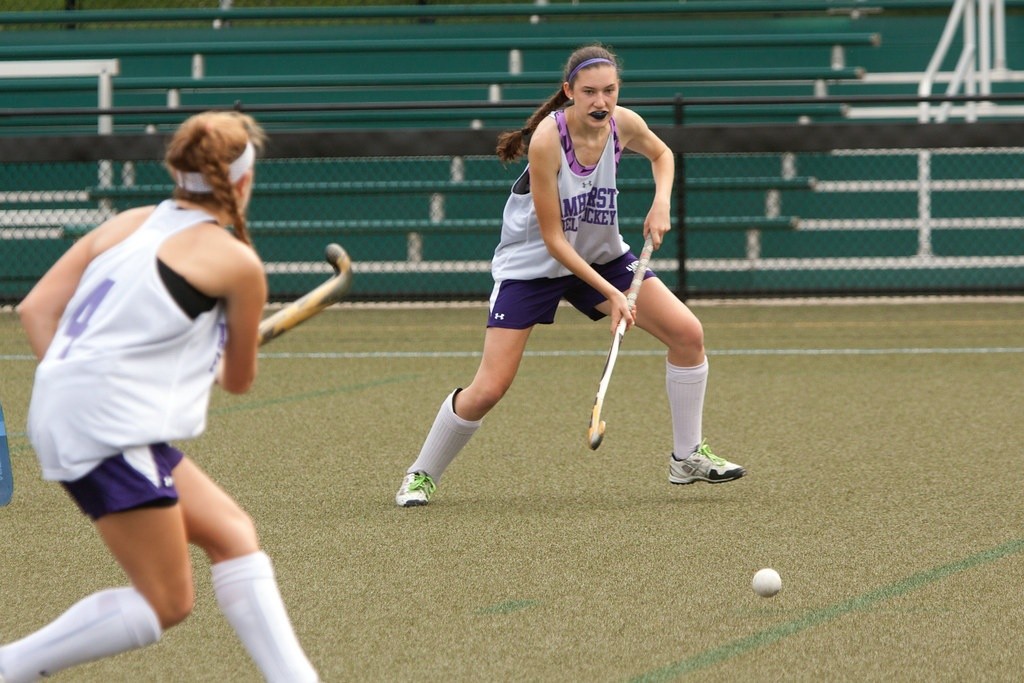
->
xmin=751 ymin=567 xmax=783 ymax=598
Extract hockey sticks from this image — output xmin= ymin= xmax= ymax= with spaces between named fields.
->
xmin=255 ymin=240 xmax=355 ymax=351
xmin=586 ymin=231 xmax=656 ymax=452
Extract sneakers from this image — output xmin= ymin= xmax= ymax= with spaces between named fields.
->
xmin=396 ymin=470 xmax=436 ymax=506
xmin=669 ymin=437 xmax=747 ymax=485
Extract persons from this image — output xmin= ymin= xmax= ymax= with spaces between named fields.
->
xmin=0 ymin=111 xmax=324 ymax=683
xmin=395 ymin=46 xmax=747 ymax=508
